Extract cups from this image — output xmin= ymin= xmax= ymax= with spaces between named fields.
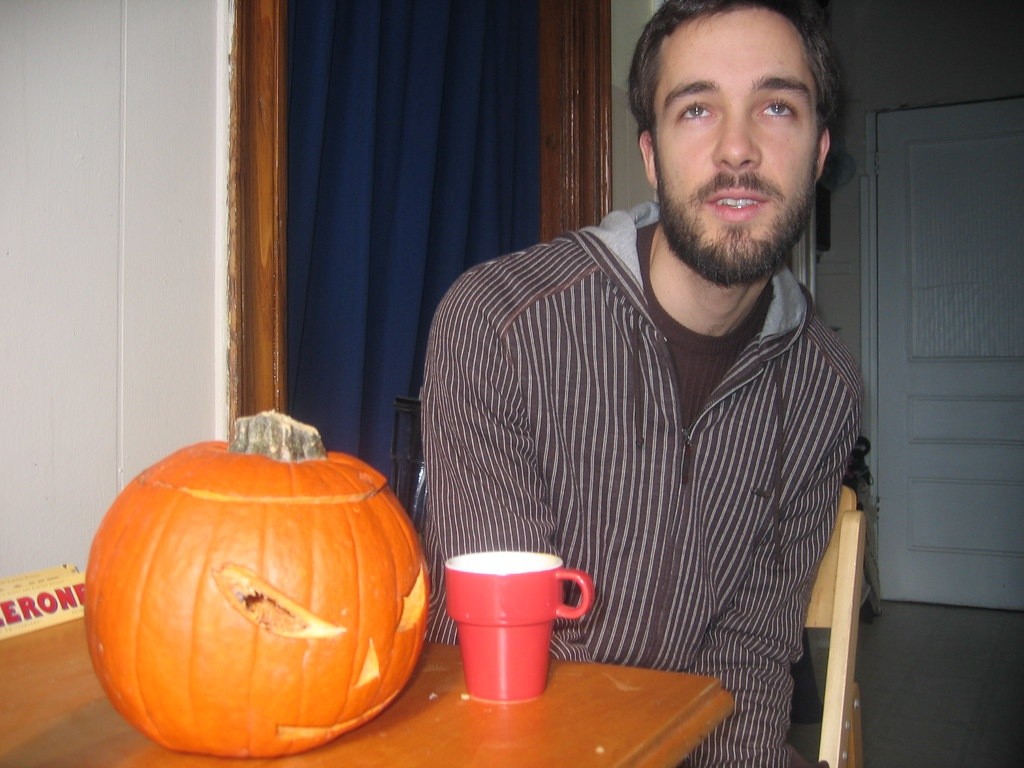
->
xmin=443 ymin=552 xmax=593 ymax=702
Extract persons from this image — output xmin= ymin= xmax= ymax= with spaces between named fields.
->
xmin=419 ymin=0 xmax=863 ymax=768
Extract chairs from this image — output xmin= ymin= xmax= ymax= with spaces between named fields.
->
xmin=796 ymin=483 xmax=867 ymax=768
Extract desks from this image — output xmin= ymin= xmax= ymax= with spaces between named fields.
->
xmin=0 ymin=616 xmax=734 ymax=767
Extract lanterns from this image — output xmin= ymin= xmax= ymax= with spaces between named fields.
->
xmin=83 ymin=412 xmax=433 ymax=760
